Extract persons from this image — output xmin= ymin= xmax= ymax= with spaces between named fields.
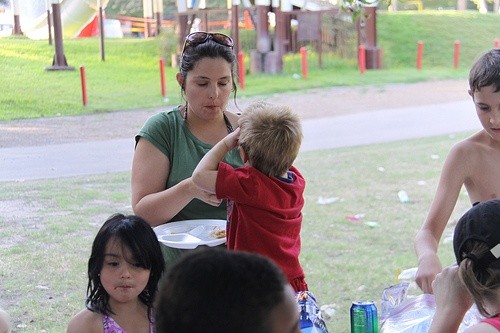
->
xmin=154 ymin=248 xmax=302 ymax=333
xmin=66 ymin=214 xmax=165 ymax=333
xmin=192 ymin=101 xmax=309 ymax=291
xmin=416 ymin=50 xmax=500 ymax=293
xmin=428 ymin=199 xmax=500 ymax=333
xmin=130 ymin=32 xmax=244 ymax=269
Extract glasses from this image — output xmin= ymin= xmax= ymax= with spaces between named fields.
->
xmin=181 ymin=31 xmax=234 ymax=54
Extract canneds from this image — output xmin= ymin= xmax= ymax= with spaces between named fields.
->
xmin=349 ymin=299 xmax=379 ymax=333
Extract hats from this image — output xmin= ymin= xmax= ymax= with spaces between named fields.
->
xmin=452 ymin=199 xmax=500 ymax=271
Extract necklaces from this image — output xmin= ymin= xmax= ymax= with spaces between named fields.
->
xmin=183 ymin=102 xmax=234 ymax=134
